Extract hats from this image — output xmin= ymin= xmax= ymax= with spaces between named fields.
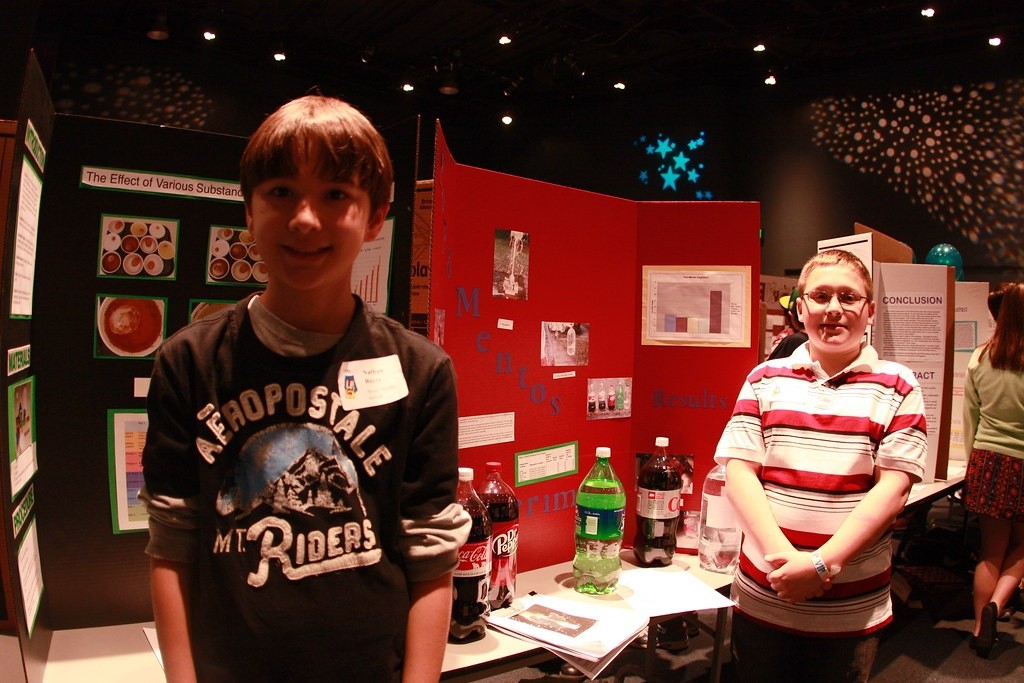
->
xmin=778 ymin=287 xmax=798 ymax=313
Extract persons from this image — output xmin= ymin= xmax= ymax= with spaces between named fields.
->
xmin=765 ymin=288 xmax=808 ymax=362
xmin=713 ymin=249 xmax=930 ymax=683
xmin=959 ymin=282 xmax=1024 ymax=658
xmin=134 ymin=96 xmax=472 ymax=683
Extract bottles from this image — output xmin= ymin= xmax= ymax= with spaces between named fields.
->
xmin=597 ymin=382 xmax=606 ymax=411
xmin=446 ymin=465 xmax=493 ymax=646
xmin=567 ymin=326 xmax=576 ymax=356
xmin=636 ymin=437 xmax=681 ymax=567
xmin=698 ymin=465 xmax=741 ymax=575
xmin=570 ymin=447 xmax=625 ymax=595
xmin=477 ymin=462 xmax=520 ymax=611
xmin=587 ymin=381 xmax=596 ymax=414
xmin=607 ymin=384 xmax=616 ymax=410
xmin=617 ymin=383 xmax=624 ymax=410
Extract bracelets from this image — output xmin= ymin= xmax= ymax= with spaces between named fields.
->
xmin=811 ymin=550 xmax=830 ymax=579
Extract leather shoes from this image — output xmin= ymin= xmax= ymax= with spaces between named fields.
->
xmin=969 ymin=602 xmax=998 ymax=659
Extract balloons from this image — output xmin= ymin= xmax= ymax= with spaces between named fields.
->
xmin=927 ymin=243 xmax=963 ymax=281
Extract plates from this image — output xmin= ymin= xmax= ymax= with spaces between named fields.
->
xmin=190 ymin=302 xmax=236 ymax=322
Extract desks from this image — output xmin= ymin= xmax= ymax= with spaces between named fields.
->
xmin=0 ymin=460 xmax=967 ymax=683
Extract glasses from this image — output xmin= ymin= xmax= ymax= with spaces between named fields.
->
xmin=800 ymin=290 xmax=871 ymax=306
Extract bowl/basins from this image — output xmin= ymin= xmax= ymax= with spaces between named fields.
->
xmin=229 ymin=228 xmax=269 ymax=285
xmin=144 ymin=223 xmax=175 ymax=283
xmin=96 ymin=297 xmax=164 ymax=356
xmin=139 ymin=235 xmax=158 ymax=254
xmin=121 ymin=218 xmax=148 ymax=277
xmin=100 ymin=217 xmax=124 ymax=274
xmin=208 ymin=226 xmax=234 ymax=281
